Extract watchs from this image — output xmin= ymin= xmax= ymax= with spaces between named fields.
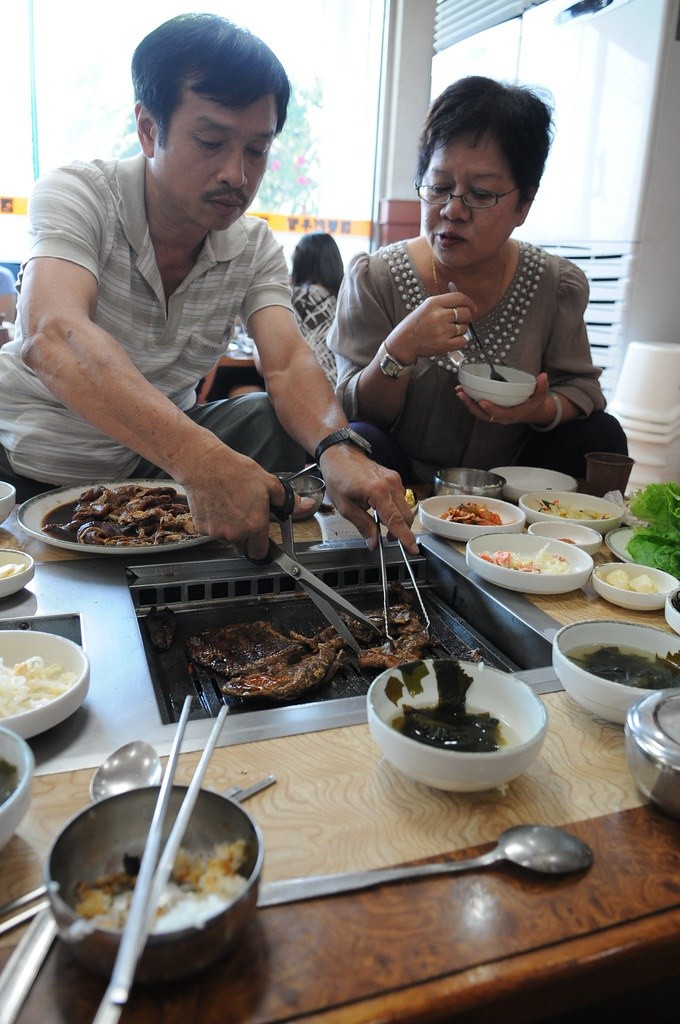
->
xmin=314 ymin=427 xmax=374 ymax=466
xmin=377 ymin=341 xmax=418 ymax=379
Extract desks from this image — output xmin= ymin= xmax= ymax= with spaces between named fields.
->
xmin=200 ymin=339 xmax=264 ymax=386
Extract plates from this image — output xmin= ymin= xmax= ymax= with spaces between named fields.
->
xmin=419 ymin=495 xmax=526 ymax=542
xmin=489 ymin=467 xmax=624 ymax=537
xmin=0 ymin=630 xmax=91 ymax=740
xmin=593 ymin=563 xmax=680 ymax=611
xmin=0 ymin=549 xmax=35 ymax=598
xmin=466 ymin=533 xmax=593 ymax=594
xmin=16 ymin=478 xmax=215 ymax=554
xmin=528 ymin=521 xmax=635 ymax=564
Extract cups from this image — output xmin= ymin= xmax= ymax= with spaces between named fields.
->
xmin=586 ymin=452 xmax=636 ymax=499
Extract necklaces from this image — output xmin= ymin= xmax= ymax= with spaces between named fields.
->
xmin=431 ymin=245 xmax=507 ymax=297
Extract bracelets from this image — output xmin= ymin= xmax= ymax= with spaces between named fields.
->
xmin=530 ymin=391 xmax=563 ymax=433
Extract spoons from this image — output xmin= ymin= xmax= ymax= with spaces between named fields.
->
xmin=448 ymin=282 xmax=509 ymax=383
xmin=0 ymin=740 xmax=163 ymax=1024
xmin=258 ymin=824 xmax=592 ymax=908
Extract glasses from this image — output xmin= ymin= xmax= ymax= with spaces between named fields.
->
xmin=413 ymin=172 xmax=518 ymax=209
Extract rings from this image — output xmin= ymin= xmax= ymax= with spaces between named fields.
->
xmin=453 ymin=308 xmax=459 ymax=322
xmin=489 ymin=416 xmax=496 ymax=424
xmin=456 ymin=324 xmax=460 ymax=336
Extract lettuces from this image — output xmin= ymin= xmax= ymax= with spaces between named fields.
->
xmin=625 ymin=482 xmax=680 ymax=582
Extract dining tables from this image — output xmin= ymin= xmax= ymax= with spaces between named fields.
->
xmin=0 ymin=518 xmax=680 ymax=1024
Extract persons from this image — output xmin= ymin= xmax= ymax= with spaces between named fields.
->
xmin=229 ymin=230 xmax=344 ymax=397
xmin=327 ymin=74 xmax=628 ymax=478
xmin=0 ymin=265 xmax=19 ymax=347
xmin=0 ymin=10 xmax=419 ymax=565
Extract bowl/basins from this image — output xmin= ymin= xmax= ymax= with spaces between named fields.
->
xmin=665 ymin=587 xmax=680 ymax=635
xmin=458 ymin=364 xmax=537 ymax=408
xmin=0 ymin=726 xmax=35 ymax=853
xmin=268 ymin=473 xmax=325 ymax=522
xmin=625 ymin=688 xmax=680 ymax=816
xmin=434 ymin=468 xmax=506 ymax=500
xmin=0 ymin=481 xmax=16 ymax=525
xmin=553 ymin=620 xmax=680 ymax=726
xmin=45 ymin=785 xmax=263 ymax=987
xmin=367 ymin=659 xmax=548 ymax=792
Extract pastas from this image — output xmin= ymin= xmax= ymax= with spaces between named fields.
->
xmin=0 ymin=656 xmax=77 ymax=718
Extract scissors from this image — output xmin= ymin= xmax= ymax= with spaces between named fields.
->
xmin=242 ymin=477 xmax=382 ymax=657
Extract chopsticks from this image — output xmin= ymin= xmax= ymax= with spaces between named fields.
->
xmin=91 ymin=695 xmax=229 ymax=1024
xmin=0 ymin=776 xmax=276 ymax=933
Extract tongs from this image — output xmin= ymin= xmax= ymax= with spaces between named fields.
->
xmin=373 ymin=506 xmax=431 ymax=639
